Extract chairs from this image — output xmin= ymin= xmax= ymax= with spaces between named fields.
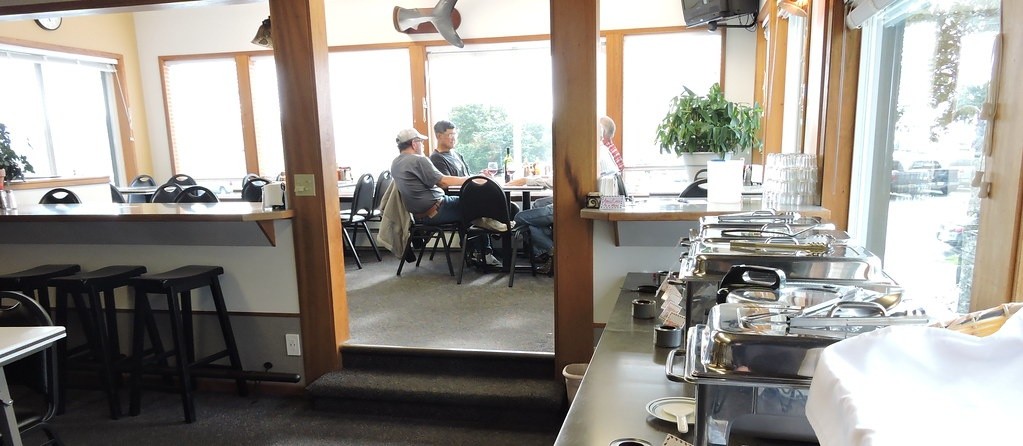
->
xmin=338 ymin=170 xmax=553 ymax=287
xmin=0 ymin=291 xmax=67 ymax=446
xmin=242 ymin=174 xmax=285 ymax=202
xmin=128 ymin=174 xmax=220 ymax=203
xmin=39 ymin=188 xmax=82 ymax=204
xmin=109 ymin=183 xmax=125 ymax=202
xmin=679 ymin=169 xmax=707 ymax=197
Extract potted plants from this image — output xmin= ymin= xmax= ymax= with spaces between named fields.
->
xmin=0 ymin=123 xmax=36 ymax=190
xmin=654 ymin=83 xmax=765 ymax=181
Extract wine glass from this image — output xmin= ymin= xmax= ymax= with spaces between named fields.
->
xmin=487 ymin=162 xmax=498 ymax=180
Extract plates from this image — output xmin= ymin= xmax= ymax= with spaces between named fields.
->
xmin=646 ymin=396 xmax=696 ymax=424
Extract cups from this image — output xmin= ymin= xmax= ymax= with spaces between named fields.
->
xmin=763 ymin=153 xmax=818 ymax=204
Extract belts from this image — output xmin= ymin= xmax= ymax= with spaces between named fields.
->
xmin=414 ymin=199 xmax=443 ymax=219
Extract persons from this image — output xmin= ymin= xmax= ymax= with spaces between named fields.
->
xmin=514 ymin=116 xmax=623 ymax=274
xmin=391 ymin=121 xmax=519 ymax=267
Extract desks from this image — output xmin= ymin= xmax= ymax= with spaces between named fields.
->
xmin=648 ymin=182 xmax=763 ymax=196
xmin=1 ymin=326 xmax=67 ymax=446
xmin=338 ymin=180 xmax=378 ymax=197
xmin=118 ymin=186 xmax=199 ymax=203
xmin=449 ymin=183 xmax=545 ymax=272
xmin=218 ymin=191 xmax=242 ymax=202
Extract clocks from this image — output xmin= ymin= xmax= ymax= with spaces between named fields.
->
xmin=34 ymin=17 xmax=62 ymax=31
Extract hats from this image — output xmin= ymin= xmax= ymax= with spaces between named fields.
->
xmin=396 ymin=128 xmax=428 ymax=144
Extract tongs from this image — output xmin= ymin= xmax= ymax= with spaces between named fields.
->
xmin=786 ymin=289 xmax=929 ymax=328
xmin=718 ymin=210 xmax=794 ymax=220
xmin=730 ymin=235 xmax=834 ymax=254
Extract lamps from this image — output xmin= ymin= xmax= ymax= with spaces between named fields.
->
xmin=250 ymin=15 xmax=273 ymax=48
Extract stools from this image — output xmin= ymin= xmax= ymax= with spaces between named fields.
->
xmin=0 ymin=265 xmax=249 ymax=423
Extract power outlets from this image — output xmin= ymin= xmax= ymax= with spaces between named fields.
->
xmin=285 ymin=334 xmax=301 ymax=356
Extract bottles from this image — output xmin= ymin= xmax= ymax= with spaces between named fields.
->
xmin=337 ymin=166 xmax=350 ymax=182
xmin=504 ymin=147 xmax=513 ymax=183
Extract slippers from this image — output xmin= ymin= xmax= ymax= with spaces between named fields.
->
xmin=472 ymin=252 xmax=501 ymax=265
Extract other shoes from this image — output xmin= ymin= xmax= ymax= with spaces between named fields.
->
xmin=533 ymin=252 xmax=553 ymax=264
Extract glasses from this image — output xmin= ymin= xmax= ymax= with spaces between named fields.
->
xmin=413 ymin=138 xmax=424 ymax=144
xmin=443 ymin=132 xmax=457 ymax=138
